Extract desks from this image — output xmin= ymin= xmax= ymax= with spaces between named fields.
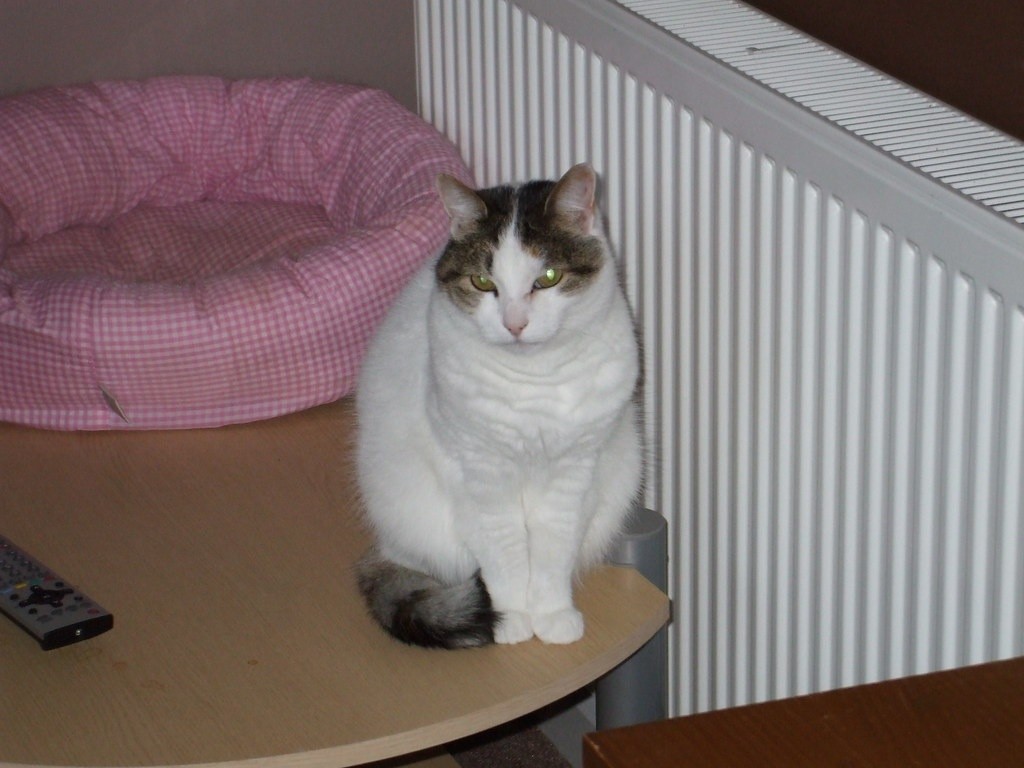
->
xmin=582 ymin=656 xmax=1024 ymax=768
xmin=0 ymin=397 xmax=669 ymax=767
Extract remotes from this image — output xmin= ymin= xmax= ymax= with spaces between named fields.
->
xmin=1 ymin=536 xmax=114 ymax=650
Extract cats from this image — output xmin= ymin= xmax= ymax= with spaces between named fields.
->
xmin=344 ymin=162 xmax=659 ymax=650
